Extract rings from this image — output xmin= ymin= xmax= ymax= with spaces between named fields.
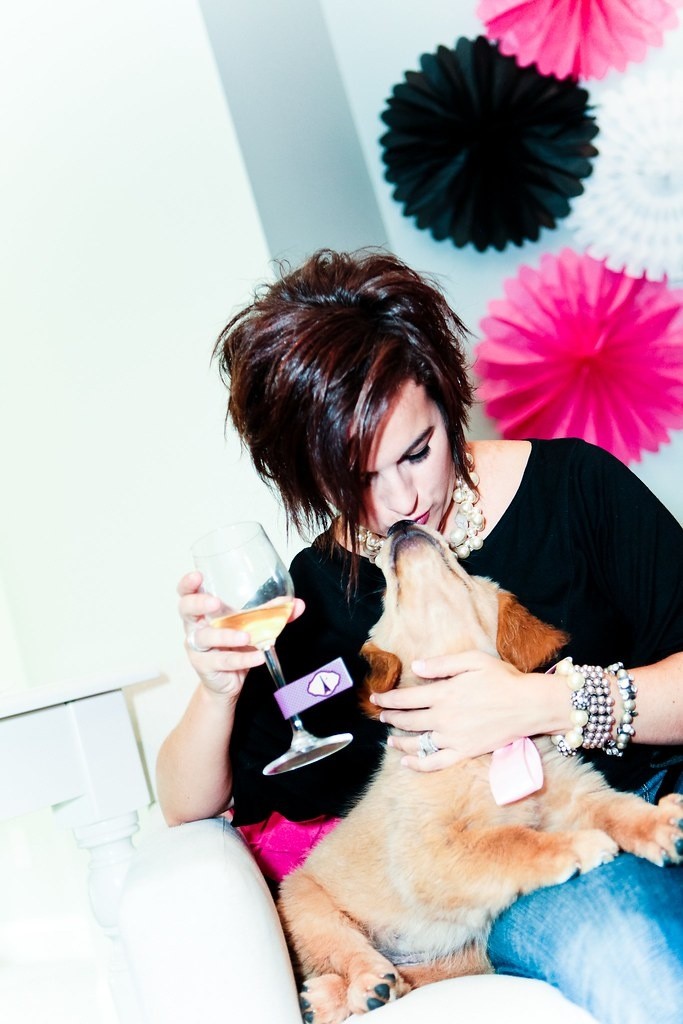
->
xmin=418 ymin=730 xmax=438 ymax=758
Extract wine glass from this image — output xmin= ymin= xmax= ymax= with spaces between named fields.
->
xmin=190 ymin=521 xmax=352 ymax=776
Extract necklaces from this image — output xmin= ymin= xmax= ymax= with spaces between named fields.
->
xmin=354 ymin=443 xmax=484 ymax=560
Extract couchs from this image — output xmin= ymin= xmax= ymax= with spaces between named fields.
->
xmin=115 ymin=815 xmax=606 ymax=1024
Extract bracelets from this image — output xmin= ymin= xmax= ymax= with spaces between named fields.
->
xmin=555 ymin=658 xmax=638 ymax=757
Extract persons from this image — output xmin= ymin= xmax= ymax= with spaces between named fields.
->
xmin=156 ymin=248 xmax=683 ymax=1024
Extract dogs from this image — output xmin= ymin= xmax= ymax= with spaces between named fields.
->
xmin=277 ymin=519 xmax=683 ymax=1024
